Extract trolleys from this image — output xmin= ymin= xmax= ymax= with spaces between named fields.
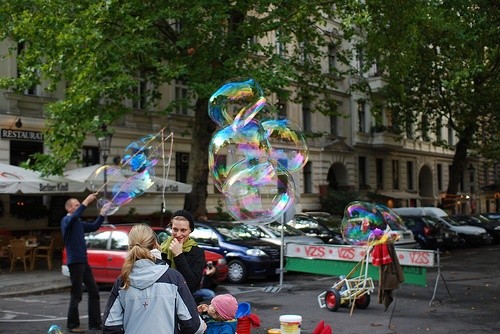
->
xmin=317 ymin=246 xmax=374 ymax=312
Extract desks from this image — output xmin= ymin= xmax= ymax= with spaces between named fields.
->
xmin=8 ymin=243 xmax=40 ymax=250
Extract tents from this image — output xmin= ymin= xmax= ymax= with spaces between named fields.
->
xmin=0 ymin=163 xmax=87 ymax=195
xmin=61 ymin=164 xmax=194 ymax=224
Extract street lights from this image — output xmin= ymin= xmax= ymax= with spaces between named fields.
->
xmin=95 ymin=122 xmax=113 ymax=227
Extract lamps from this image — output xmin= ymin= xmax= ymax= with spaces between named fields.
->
xmin=9 ymin=113 xmax=22 ymax=130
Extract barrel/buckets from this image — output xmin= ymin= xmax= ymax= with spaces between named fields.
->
xmin=279 ymin=315 xmax=302 ymax=334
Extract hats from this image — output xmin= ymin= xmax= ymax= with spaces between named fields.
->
xmin=211 ymin=294 xmax=237 ymax=320
xmin=170 ymin=209 xmax=195 ymax=233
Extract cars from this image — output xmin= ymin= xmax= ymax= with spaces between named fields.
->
xmin=162 ymin=210 xmax=348 ymax=285
xmin=391 ymin=206 xmax=500 ymax=255
xmin=59 ymin=223 xmax=228 ymax=292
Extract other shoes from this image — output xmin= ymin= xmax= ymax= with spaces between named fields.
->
xmin=69 ymin=327 xmax=85 ymax=333
xmin=89 ymin=324 xmax=102 ymax=330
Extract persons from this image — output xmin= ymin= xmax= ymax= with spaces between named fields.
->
xmin=161 ymin=209 xmax=207 ymax=298
xmin=101 ymin=223 xmax=207 ymax=334
xmin=61 ymin=192 xmax=113 ymax=332
xmin=197 ymin=293 xmax=238 ymax=334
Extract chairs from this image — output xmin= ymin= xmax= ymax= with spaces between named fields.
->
xmin=10 ymin=239 xmax=31 ymax=273
xmin=30 ymin=236 xmax=56 ymax=271
